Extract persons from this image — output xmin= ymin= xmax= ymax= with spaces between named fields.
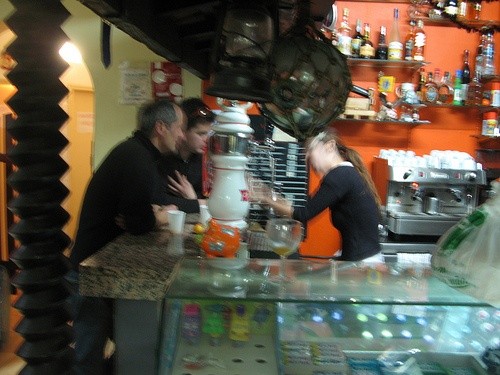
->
xmin=158 ymin=98 xmax=215 ymax=214
xmin=68 ymin=99 xmax=185 ymax=375
xmin=243 ymin=131 xmax=384 ymax=264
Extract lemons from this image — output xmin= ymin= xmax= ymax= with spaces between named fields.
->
xmin=193 ymin=224 xmax=205 ymax=233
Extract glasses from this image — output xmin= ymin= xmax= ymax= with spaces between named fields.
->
xmin=187 ymin=106 xmax=218 ymax=123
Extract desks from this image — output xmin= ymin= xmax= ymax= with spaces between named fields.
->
xmin=154 ymin=255 xmax=500 ymax=375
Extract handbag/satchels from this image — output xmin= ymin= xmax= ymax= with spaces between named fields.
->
xmin=429 ymin=197 xmax=500 ymax=310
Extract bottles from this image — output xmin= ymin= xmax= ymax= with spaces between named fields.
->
xmin=329 ymin=29 xmax=338 ymax=46
xmin=361 ymin=22 xmax=373 ymax=59
xmin=437 ymin=71 xmax=454 ymax=104
xmin=404 ymin=19 xmax=416 ymax=61
xmin=414 ymin=19 xmax=426 ymax=61
xmin=433 ymin=68 xmax=441 ymax=86
xmin=415 ymin=67 xmax=427 ymax=103
xmin=429 ymin=0 xmax=481 ymax=21
xmin=462 ymin=20 xmax=500 ymax=136
xmin=453 ymin=70 xmax=462 ymax=105
xmin=376 ymin=25 xmax=387 ymax=60
xmin=351 ymin=19 xmax=363 ymax=58
xmin=387 ymin=8 xmax=404 ymax=60
xmin=338 ymin=8 xmax=352 ymax=58
xmin=424 ymin=72 xmax=438 ymax=104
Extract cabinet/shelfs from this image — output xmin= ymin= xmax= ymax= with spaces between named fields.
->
xmin=408 ymin=15 xmax=500 ymax=110
xmin=476 ymin=74 xmax=500 ymax=146
xmin=334 ymin=55 xmax=432 ymax=125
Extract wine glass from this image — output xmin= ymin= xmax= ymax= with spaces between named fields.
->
xmin=265 ymin=218 xmax=302 ymax=286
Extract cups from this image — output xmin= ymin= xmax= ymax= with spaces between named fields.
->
xmin=379 ymin=149 xmax=477 ymax=170
xmin=396 ymin=83 xmax=415 ymax=100
xmin=167 ymin=209 xmax=185 ymax=235
xmin=200 ymin=205 xmax=211 ymax=227
